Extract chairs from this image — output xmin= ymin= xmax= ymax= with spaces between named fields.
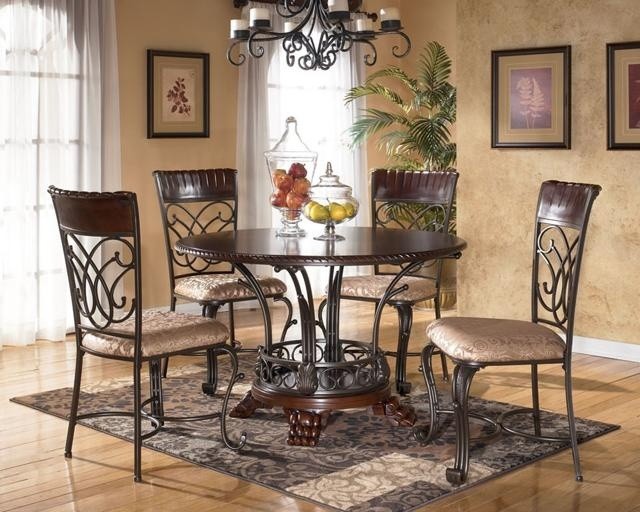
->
xmin=44 ymin=185 xmax=247 ymax=482
xmin=416 ymin=176 xmax=599 ymax=486
xmin=154 ymin=168 xmax=298 ymax=391
xmin=320 ymin=168 xmax=460 ymax=396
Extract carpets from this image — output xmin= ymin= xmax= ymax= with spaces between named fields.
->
xmin=11 ymin=349 xmax=620 ymax=510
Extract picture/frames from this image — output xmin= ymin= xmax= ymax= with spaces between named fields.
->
xmin=491 ymin=43 xmax=571 ymax=154
xmin=144 ymin=48 xmax=210 ymax=141
xmin=604 ymin=41 xmax=640 ymax=150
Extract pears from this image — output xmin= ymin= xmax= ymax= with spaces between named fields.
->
xmin=305 ymin=197 xmax=354 ymax=221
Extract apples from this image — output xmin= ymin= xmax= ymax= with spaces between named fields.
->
xmin=271 ymin=163 xmax=311 ymax=220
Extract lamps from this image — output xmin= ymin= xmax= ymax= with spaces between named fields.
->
xmin=221 ymin=1 xmax=410 ymax=70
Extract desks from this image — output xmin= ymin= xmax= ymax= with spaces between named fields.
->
xmin=174 ymin=225 xmax=467 ymax=444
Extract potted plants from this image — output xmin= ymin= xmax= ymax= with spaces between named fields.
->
xmin=340 ymin=40 xmax=461 ymax=310
